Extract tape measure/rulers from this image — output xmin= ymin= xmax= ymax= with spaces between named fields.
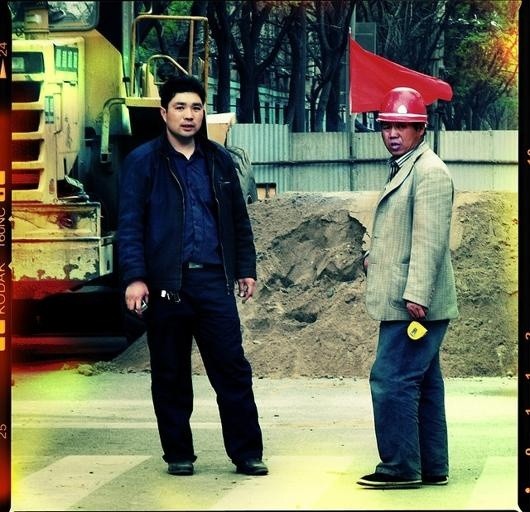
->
xmin=407 ymin=320 xmax=428 ymax=340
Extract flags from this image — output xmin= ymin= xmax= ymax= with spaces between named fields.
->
xmin=349 ymin=37 xmax=454 ymax=113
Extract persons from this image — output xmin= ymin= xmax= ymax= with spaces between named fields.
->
xmin=120 ymin=73 xmax=270 ymax=477
xmin=354 ymin=86 xmax=463 ymax=489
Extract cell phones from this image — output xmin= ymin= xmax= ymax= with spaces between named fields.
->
xmin=135 ymin=299 xmax=147 ymax=315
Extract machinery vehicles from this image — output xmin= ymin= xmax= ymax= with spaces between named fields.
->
xmin=11 ymin=1 xmax=258 ymax=299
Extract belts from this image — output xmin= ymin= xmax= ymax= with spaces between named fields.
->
xmin=184 ymin=260 xmax=224 ymax=272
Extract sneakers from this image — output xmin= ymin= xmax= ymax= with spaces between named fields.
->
xmin=355 ymin=472 xmax=450 ymax=489
xmin=235 ymin=460 xmax=270 ymax=476
xmin=166 ymin=459 xmax=195 ymax=477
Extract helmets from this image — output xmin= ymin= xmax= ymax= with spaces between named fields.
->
xmin=374 ymin=85 xmax=429 ymax=126
